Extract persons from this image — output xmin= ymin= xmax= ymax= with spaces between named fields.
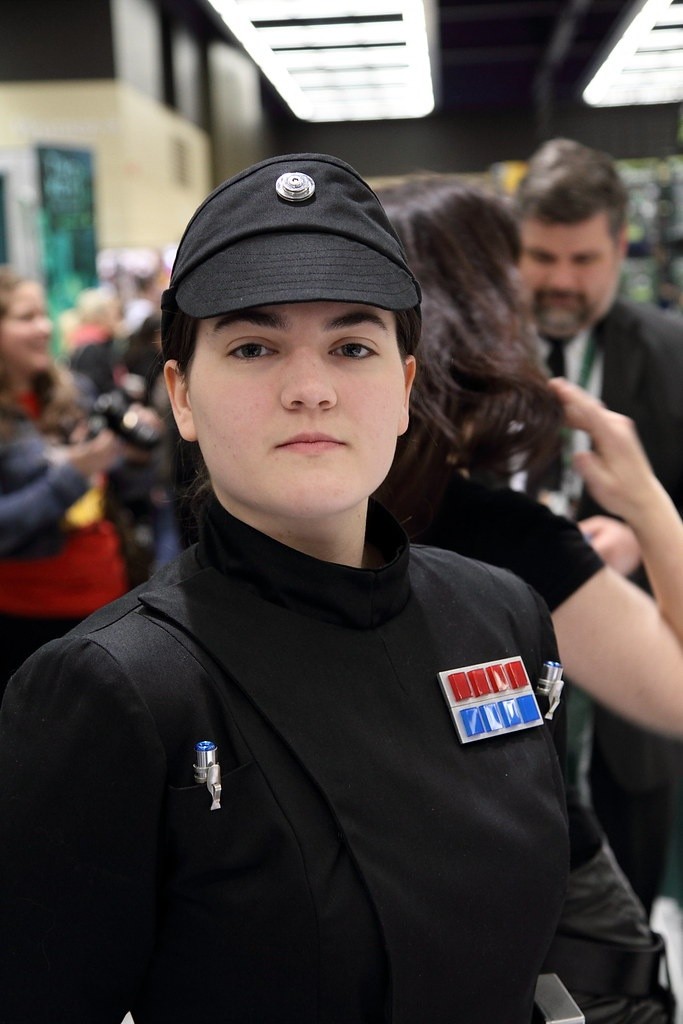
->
xmin=0 ymin=153 xmax=677 ymax=1023
xmin=374 ymin=172 xmax=683 ymax=744
xmin=511 ymin=140 xmax=682 ymax=927
xmin=0 ymin=245 xmax=171 ymax=686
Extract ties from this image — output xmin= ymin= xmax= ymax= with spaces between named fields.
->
xmin=526 ymin=334 xmax=575 ymax=498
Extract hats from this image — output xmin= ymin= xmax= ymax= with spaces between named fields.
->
xmin=161 ymin=153 xmax=422 ymax=319
